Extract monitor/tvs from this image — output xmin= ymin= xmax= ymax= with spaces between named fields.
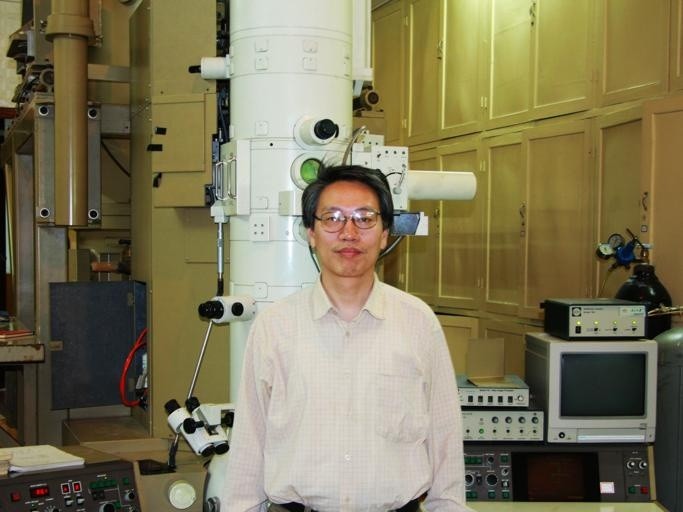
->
xmin=525 ymin=331 xmax=658 ymax=444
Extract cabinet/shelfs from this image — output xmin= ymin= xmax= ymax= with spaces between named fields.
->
xmin=367 ymin=94 xmax=680 ymax=322
xmin=420 ymin=302 xmax=546 ymax=396
xmin=369 ymin=0 xmax=682 ymax=148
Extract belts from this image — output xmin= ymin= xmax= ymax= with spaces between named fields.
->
xmin=275 ymin=501 xmax=422 ymax=511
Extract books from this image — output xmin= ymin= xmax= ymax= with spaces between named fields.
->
xmin=0 ymin=442 xmax=86 ymax=473
xmin=0 ymin=327 xmax=36 ymax=340
xmin=466 ymin=374 xmax=521 ymax=389
xmin=0 ymin=448 xmax=15 ymax=478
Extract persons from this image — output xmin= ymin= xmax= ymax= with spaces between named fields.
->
xmin=218 ymin=165 xmax=475 ymax=512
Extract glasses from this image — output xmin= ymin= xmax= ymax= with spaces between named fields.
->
xmin=315 ymin=207 xmax=383 ymax=233
